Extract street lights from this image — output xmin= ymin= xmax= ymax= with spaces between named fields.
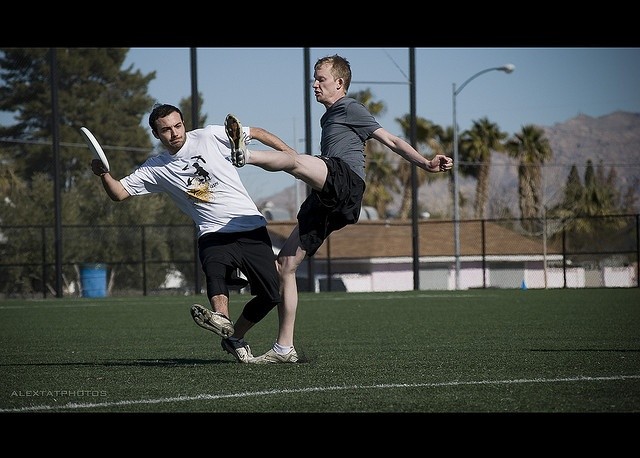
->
xmin=451 ymin=64 xmax=514 ymax=290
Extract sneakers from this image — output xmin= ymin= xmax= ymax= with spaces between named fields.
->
xmin=222 ymin=338 xmax=254 ymax=364
xmin=190 ymin=304 xmax=235 ymax=337
xmin=249 ymin=348 xmax=299 ymax=364
xmin=225 ymin=114 xmax=251 ymax=168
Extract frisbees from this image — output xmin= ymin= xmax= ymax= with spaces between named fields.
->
xmin=80 ymin=126 xmax=110 ymax=172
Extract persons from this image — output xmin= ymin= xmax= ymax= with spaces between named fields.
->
xmin=89 ymin=104 xmax=301 ymax=365
xmin=222 ymin=54 xmax=454 ymax=363
